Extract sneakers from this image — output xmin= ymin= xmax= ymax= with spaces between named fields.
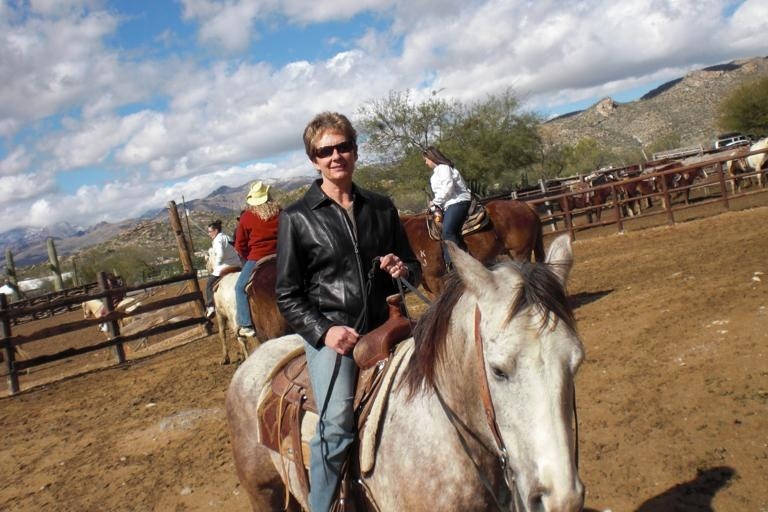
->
xmin=205 ymin=306 xmax=216 ymax=320
xmin=238 ymin=326 xmax=255 ymax=338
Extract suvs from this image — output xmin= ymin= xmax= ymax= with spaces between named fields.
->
xmin=711 ymin=131 xmax=757 ymax=151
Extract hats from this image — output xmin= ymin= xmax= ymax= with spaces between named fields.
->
xmin=246 ymin=181 xmax=272 ymax=206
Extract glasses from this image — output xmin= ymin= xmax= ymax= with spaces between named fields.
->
xmin=316 ymin=141 xmax=354 ymax=159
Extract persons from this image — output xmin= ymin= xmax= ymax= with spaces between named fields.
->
xmin=203 ymin=219 xmax=242 ymax=321
xmin=226 ymin=209 xmax=245 ymax=247
xmin=96 ymin=270 xmax=126 ymax=333
xmin=420 ymin=145 xmax=473 ymax=275
xmin=271 ymin=109 xmax=425 ymax=511
xmin=230 ymin=179 xmax=284 ymax=340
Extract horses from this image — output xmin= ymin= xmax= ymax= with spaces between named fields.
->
xmin=199 ymin=247 xmax=245 ymax=366
xmin=223 ymin=232 xmax=588 ymax=512
xmin=721 ymin=137 xmax=768 ymax=194
xmin=557 ymin=159 xmax=713 ymax=231
xmin=394 ymin=198 xmax=547 ymax=299
xmin=82 ymin=296 xmax=149 ymax=363
xmin=243 ymin=256 xmax=296 ymax=344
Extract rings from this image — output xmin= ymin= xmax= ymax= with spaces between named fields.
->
xmin=389 ymin=260 xmax=396 ymax=266
